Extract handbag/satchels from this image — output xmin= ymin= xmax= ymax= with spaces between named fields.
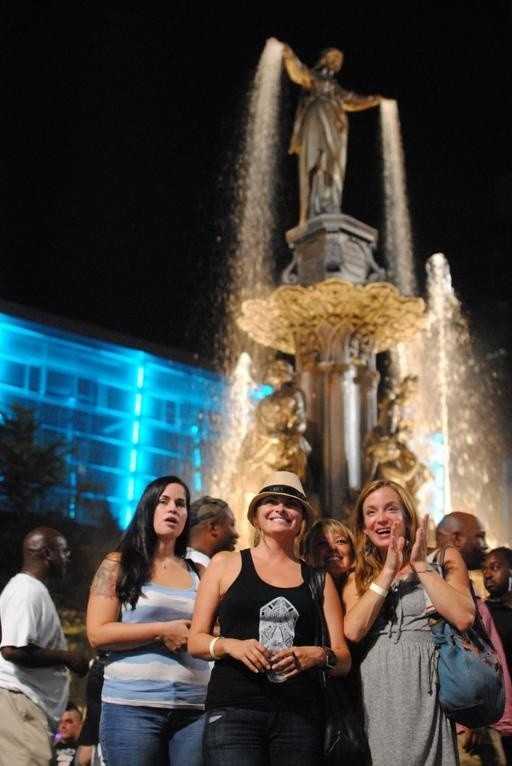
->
xmin=324 ymin=680 xmax=372 ymax=766
xmin=75 ymin=661 xmax=105 ymax=746
xmin=432 ymin=543 xmax=505 ymax=728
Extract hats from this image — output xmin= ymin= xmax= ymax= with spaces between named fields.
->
xmin=247 ymin=471 xmax=314 ymax=536
xmin=187 ymin=495 xmax=227 ymax=528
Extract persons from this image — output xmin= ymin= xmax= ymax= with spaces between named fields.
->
xmin=343 ymin=480 xmax=475 ymax=765
xmin=1 ymin=527 xmax=89 ymax=764
xmin=436 ymin=512 xmax=512 ymax=766
xmin=86 ymin=475 xmax=211 ymax=766
xmin=47 ymin=704 xmax=83 ymax=765
xmin=362 ymin=375 xmax=433 ymax=489
xmin=184 ymin=495 xmax=359 ymax=586
xmin=282 ymin=42 xmax=384 ymax=225
xmin=241 ymin=360 xmax=312 ymax=479
xmin=188 ymin=471 xmax=351 ymax=766
